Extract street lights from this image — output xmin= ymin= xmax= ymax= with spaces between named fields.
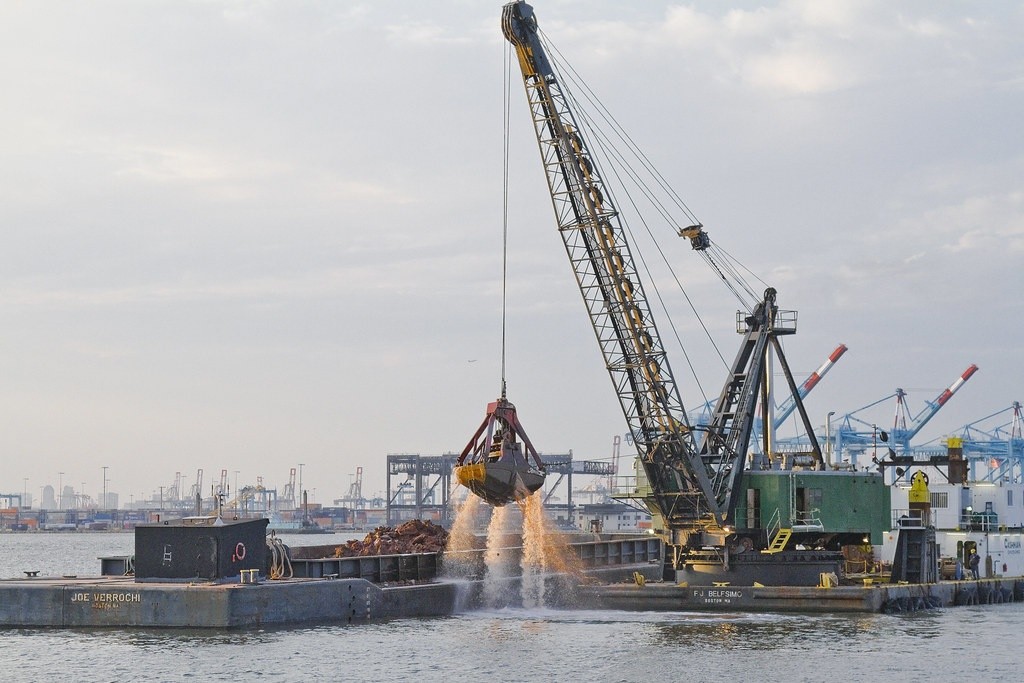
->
xmin=40 ymin=486 xmax=45 ymax=505
xmin=160 ymin=485 xmax=165 ymax=508
xmin=298 ymin=464 xmax=305 ymax=509
xmin=234 ymin=471 xmax=240 ymax=513
xmin=130 ymin=495 xmax=133 ymax=510
xmin=81 ymin=482 xmax=84 ymax=507
xmin=58 ymin=472 xmax=63 ymax=509
xmin=348 ymin=473 xmax=354 ymax=509
xmin=181 ymin=476 xmax=186 ymax=504
xmin=313 ymin=487 xmax=316 ymax=503
xmin=102 ymin=467 xmax=109 ymax=506
xmin=23 ymin=477 xmax=28 ymax=506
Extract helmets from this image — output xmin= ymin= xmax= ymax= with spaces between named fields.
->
xmin=971 ymin=549 xmax=975 ymax=554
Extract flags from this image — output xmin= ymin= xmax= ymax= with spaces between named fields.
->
xmin=990 ymin=458 xmax=999 ymax=468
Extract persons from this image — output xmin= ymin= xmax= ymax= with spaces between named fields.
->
xmin=969 ymin=549 xmax=980 ymax=580
